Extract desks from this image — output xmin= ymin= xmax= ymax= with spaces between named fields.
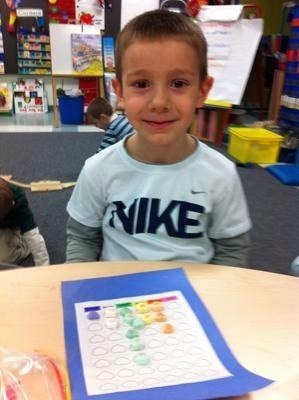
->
xmin=0 ymin=261 xmax=299 ymax=400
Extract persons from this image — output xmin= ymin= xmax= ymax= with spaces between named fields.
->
xmin=0 ymin=173 xmax=50 ymax=266
xmin=87 ymin=98 xmax=134 ymax=154
xmin=64 ymin=11 xmax=253 ymax=266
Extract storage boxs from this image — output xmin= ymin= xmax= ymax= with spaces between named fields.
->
xmin=228 ymin=127 xmax=285 ymax=165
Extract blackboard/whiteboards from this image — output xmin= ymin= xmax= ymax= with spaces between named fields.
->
xmin=49 ymin=23 xmax=102 ymax=76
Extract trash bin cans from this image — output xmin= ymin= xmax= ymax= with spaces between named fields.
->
xmin=58 ymin=94 xmax=84 ymax=124
